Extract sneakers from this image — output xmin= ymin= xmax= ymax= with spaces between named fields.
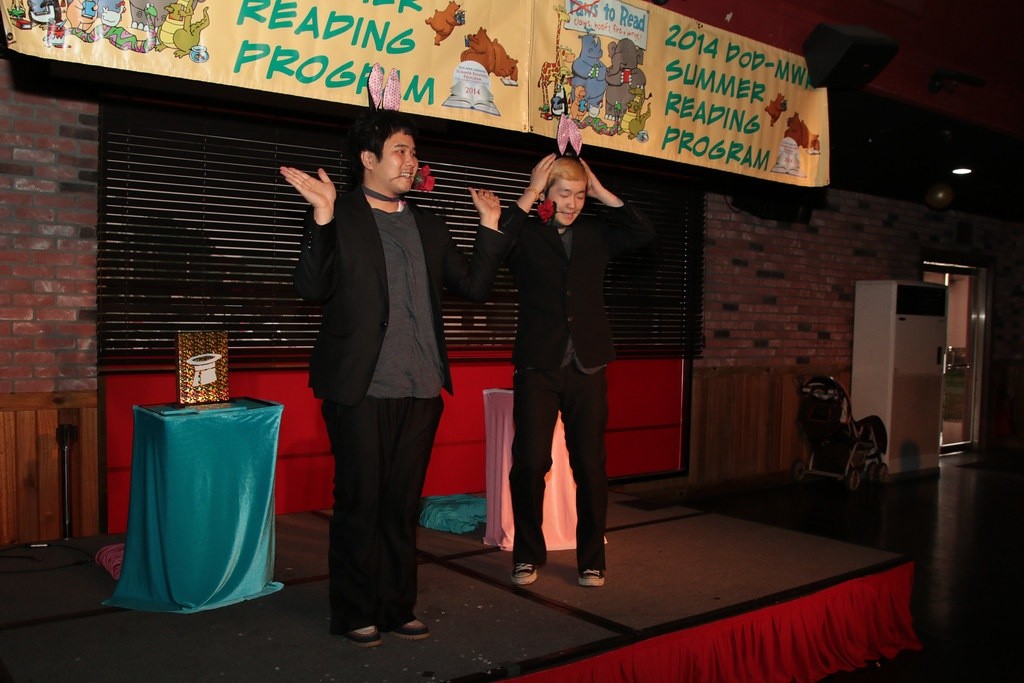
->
xmin=578 ymin=567 xmax=605 ymax=586
xmin=393 ymin=618 xmax=430 ymax=639
xmin=345 ymin=624 xmax=383 ymax=647
xmin=511 ymin=561 xmax=538 ymax=586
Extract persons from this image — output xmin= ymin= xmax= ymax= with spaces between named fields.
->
xmin=280 ymin=108 xmax=504 ymax=647
xmin=497 ymin=153 xmax=633 ymax=586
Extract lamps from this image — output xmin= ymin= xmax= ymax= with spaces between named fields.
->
xmin=800 ymin=23 xmax=901 ymax=93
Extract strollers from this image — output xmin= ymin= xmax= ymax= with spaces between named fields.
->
xmin=790 ymin=373 xmax=888 ymax=491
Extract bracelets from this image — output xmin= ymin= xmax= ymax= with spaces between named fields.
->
xmin=524 ymin=186 xmax=539 ymax=201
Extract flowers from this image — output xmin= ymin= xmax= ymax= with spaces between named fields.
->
xmin=388 ymin=165 xmax=435 ymax=193
xmin=536 ymin=200 xmax=599 ymax=233
